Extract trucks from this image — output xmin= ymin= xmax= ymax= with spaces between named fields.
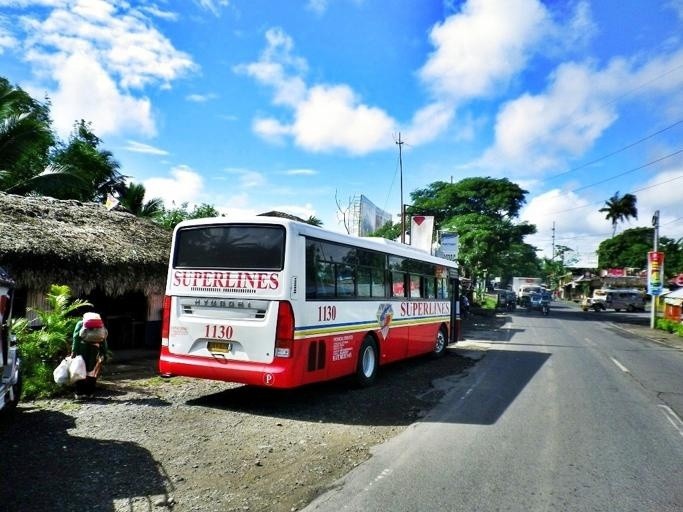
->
xmin=581 ymin=289 xmax=646 ymax=313
xmin=511 ymin=276 xmax=543 ymax=306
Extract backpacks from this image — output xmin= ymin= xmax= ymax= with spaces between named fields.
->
xmin=79 ymin=311 xmax=108 ymax=344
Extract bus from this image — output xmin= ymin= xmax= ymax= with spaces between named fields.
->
xmin=160 ymin=216 xmax=472 ymax=393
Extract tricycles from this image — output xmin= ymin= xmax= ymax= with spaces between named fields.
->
xmin=527 ymin=294 xmax=549 ymax=316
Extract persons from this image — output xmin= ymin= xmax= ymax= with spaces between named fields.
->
xmin=498 ymin=290 xmax=516 ymax=312
xmin=541 ymin=288 xmax=551 ymax=316
xmin=70 ymin=321 xmax=108 ymax=403
xmin=460 ymin=294 xmax=470 ymax=322
